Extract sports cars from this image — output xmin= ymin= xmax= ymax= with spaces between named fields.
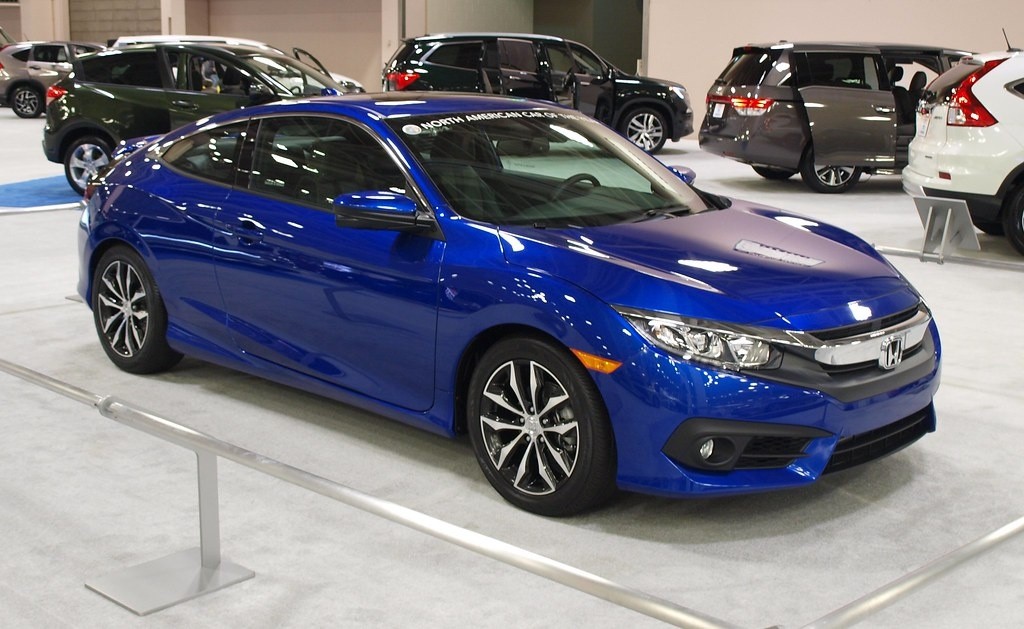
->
xmin=70 ymin=86 xmax=944 ymax=519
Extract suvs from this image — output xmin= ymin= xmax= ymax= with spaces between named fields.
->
xmin=900 ymin=47 xmax=1024 ymax=250
xmin=107 ymin=35 xmax=367 ymax=103
xmin=697 ymin=37 xmax=991 ymax=195
xmin=376 ymin=31 xmax=694 ymax=155
xmin=41 ymin=38 xmax=366 ymax=199
xmin=1 ymin=40 xmax=135 ymax=120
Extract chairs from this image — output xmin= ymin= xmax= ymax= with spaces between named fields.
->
xmin=426 ymin=130 xmax=502 ymax=220
xmin=888 ymin=66 xmax=926 ymax=123
xmin=296 ymin=147 xmax=371 ymax=206
